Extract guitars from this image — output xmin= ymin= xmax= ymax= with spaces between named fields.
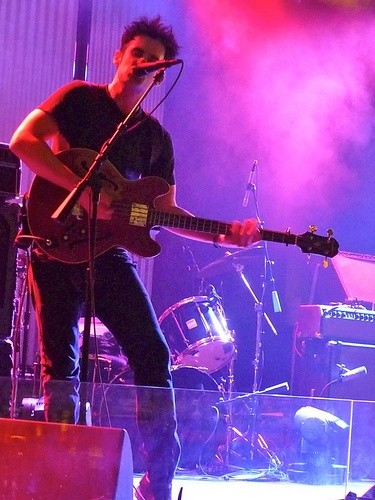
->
xmin=26 ymin=147 xmax=341 ymax=269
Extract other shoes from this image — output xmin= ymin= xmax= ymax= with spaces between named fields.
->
xmin=135 ymin=473 xmax=156 ymax=500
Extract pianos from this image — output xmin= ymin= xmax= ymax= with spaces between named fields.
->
xmin=297 ymin=304 xmax=375 ymax=349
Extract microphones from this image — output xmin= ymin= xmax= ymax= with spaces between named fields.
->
xmin=335 ymin=366 xmax=367 ymax=384
xmin=243 ymin=161 xmax=257 ymax=207
xmin=133 ymin=58 xmax=182 ymax=77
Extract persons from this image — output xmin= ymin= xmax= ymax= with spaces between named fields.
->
xmin=8 ymin=13 xmax=262 ymax=500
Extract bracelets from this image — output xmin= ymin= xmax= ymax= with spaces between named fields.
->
xmin=214 ymin=234 xmax=222 ymax=248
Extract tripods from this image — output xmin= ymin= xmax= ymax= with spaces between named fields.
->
xmin=217 ymin=256 xmax=288 ymax=476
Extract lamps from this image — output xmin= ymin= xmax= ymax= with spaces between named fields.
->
xmin=284 ymin=404 xmax=350 ymax=485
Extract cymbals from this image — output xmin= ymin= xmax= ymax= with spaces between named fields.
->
xmin=197 ymin=245 xmax=267 ymax=279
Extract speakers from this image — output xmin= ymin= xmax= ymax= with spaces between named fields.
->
xmin=297 ymin=339 xmax=375 ymax=482
xmin=0 ymin=190 xmax=34 ymax=378
xmin=0 ymin=417 xmax=134 ymax=500
xmin=301 ymin=251 xmax=375 ymax=311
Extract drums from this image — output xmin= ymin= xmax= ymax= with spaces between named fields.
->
xmin=97 ymin=363 xmax=227 ymax=472
xmin=79 ymin=319 xmax=130 ymax=369
xmin=158 ymin=295 xmax=236 ymax=374
